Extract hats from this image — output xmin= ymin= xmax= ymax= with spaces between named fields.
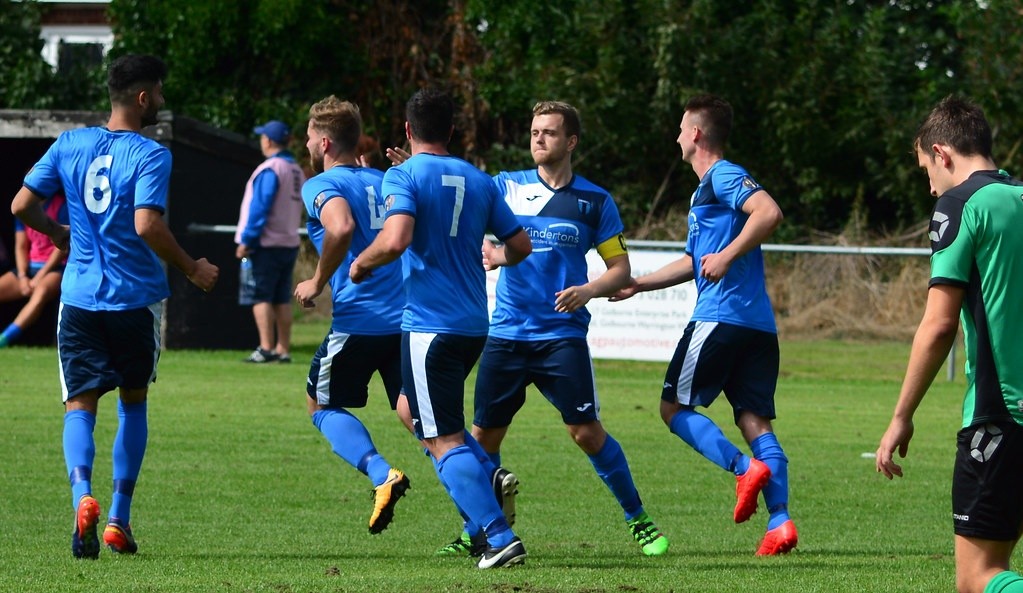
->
xmin=252 ymin=120 xmax=291 ymax=144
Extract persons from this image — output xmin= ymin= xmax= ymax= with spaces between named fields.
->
xmin=0 ymin=191 xmax=69 ymax=347
xmin=234 ymin=122 xmax=307 ymax=363
xmin=891 ymin=92 xmax=1023 ymax=593
xmin=610 ymin=93 xmax=798 ymax=555
xmin=11 ymin=53 xmax=220 ymax=559
xmin=293 ymin=88 xmax=669 ymax=570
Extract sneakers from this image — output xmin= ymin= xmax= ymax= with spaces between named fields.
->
xmin=101 ymin=516 xmax=138 ymax=555
xmin=368 ymin=468 xmax=412 ymax=536
xmin=432 ymin=532 xmax=478 ymax=559
xmin=625 ymin=511 xmax=670 ymax=557
xmin=489 ymin=465 xmax=520 ymax=528
xmin=71 ymin=495 xmax=100 ymax=560
xmin=732 ymin=456 xmax=773 ymax=524
xmin=245 ymin=348 xmax=291 ymax=364
xmin=752 ymin=519 xmax=799 ymax=557
xmin=470 ymin=535 xmax=528 ymax=569
